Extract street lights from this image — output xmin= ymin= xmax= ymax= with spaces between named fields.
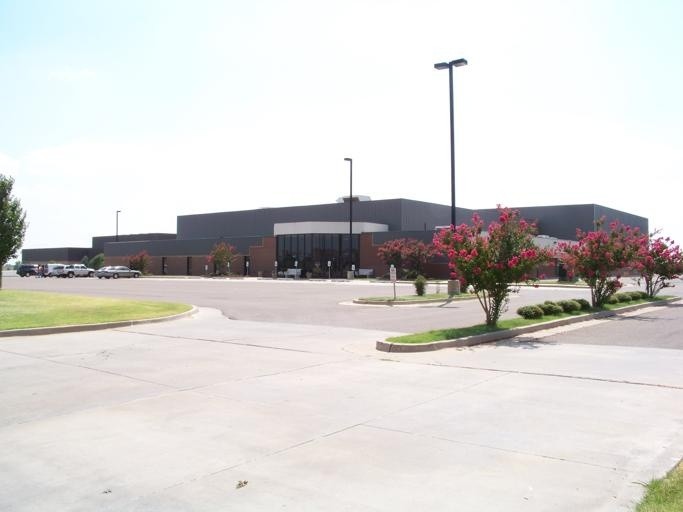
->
xmin=433 ymin=58 xmax=469 ymax=232
xmin=343 ymin=157 xmax=355 ymax=280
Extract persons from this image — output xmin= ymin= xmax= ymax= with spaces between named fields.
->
xmin=35 ymin=264 xmax=48 ymax=279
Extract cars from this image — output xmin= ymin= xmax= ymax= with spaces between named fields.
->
xmin=16 ymin=263 xmax=142 ymax=279
